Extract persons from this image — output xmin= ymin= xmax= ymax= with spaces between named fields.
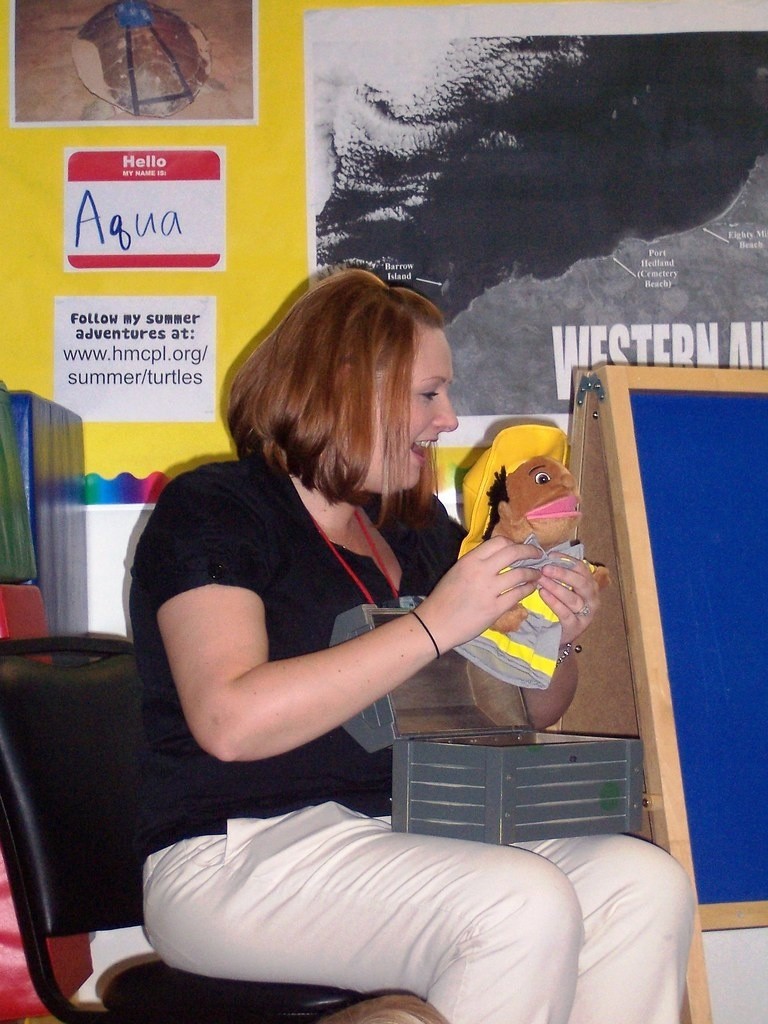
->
xmin=129 ymin=270 xmax=693 ymax=1024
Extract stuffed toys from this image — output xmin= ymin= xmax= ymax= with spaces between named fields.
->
xmin=450 ymin=423 xmax=611 ymax=691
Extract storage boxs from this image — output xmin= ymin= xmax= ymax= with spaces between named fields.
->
xmin=330 ymin=595 xmax=645 ymax=845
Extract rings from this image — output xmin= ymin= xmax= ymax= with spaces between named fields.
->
xmin=578 ymin=606 xmax=590 ymax=615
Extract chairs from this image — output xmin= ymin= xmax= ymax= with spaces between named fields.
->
xmin=0 ymin=635 xmax=372 ymax=1024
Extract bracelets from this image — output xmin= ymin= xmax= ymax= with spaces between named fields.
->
xmin=557 ymin=644 xmax=572 ymax=664
xmin=412 ymin=612 xmax=441 ymax=660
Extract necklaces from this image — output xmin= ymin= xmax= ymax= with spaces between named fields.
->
xmin=322 ymin=520 xmax=355 ymax=550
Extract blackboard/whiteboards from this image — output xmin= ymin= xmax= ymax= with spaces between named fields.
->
xmin=593 ymin=367 xmax=768 ymax=939
